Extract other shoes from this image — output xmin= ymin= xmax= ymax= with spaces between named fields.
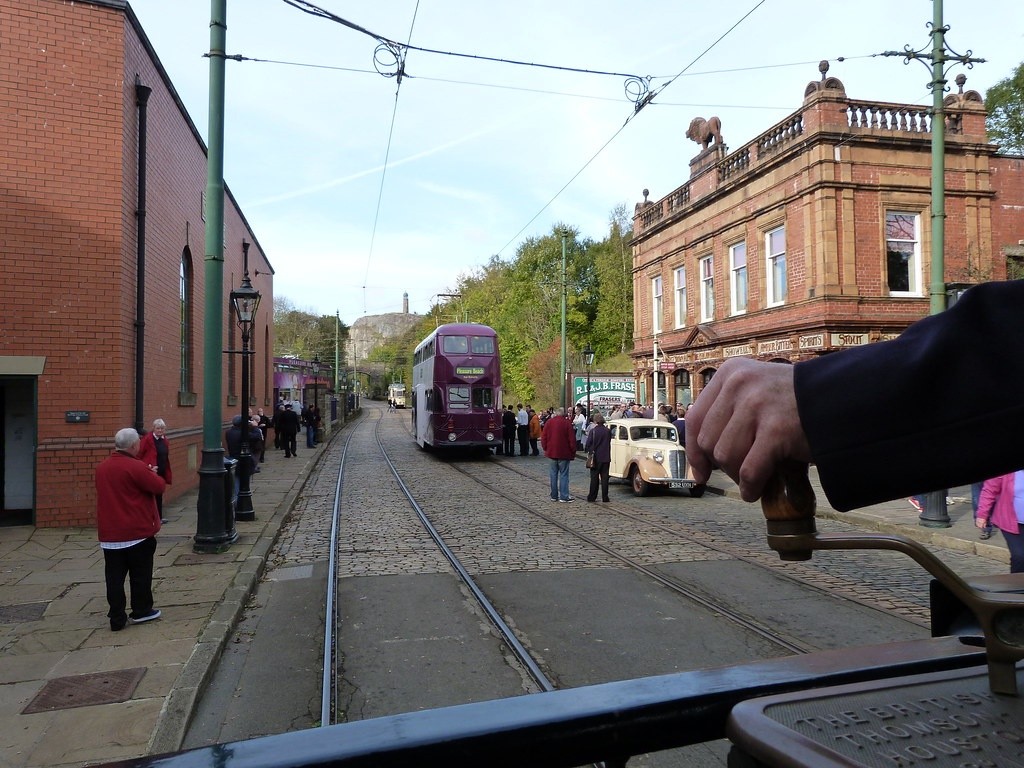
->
xmin=979 ymin=531 xmax=989 ymax=540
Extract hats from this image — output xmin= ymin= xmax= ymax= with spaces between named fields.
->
xmin=284 ymin=403 xmax=292 ymax=409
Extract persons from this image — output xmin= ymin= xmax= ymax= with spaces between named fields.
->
xmin=134 ymin=418 xmax=173 ymax=525
xmin=585 ymin=413 xmax=612 ymax=503
xmin=976 ymin=468 xmax=1024 ymax=574
xmin=495 ymin=403 xmax=693 ymax=457
xmin=387 ymin=399 xmax=397 ymax=414
xmin=541 ymin=408 xmax=577 ymax=502
xmin=685 ymin=279 xmax=1024 ymax=512
xmin=226 ymin=396 xmax=321 ymax=507
xmin=95 ymin=428 xmax=167 ymax=631
xmin=908 ymin=481 xmax=998 ymax=539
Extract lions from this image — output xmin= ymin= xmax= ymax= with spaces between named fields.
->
xmin=685 ymin=117 xmax=723 ymax=152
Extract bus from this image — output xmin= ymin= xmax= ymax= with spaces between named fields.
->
xmin=412 ymin=323 xmax=503 ymax=455
xmin=388 ymin=383 xmax=406 ymax=408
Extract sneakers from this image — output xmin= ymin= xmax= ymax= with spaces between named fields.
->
xmin=550 ymin=497 xmax=558 ymax=502
xmin=127 ymin=609 xmax=161 ymax=623
xmin=559 ymin=497 xmax=576 ymax=503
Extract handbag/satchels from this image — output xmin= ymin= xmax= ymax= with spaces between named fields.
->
xmin=585 ymin=429 xmax=597 ymax=469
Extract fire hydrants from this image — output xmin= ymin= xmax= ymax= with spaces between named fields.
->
xmin=919 ymin=489 xmax=952 ymax=528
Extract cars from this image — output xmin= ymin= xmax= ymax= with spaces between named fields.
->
xmin=599 ymin=418 xmax=707 ymax=498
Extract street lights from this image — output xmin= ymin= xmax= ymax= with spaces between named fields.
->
xmin=582 ymin=342 xmax=595 ymax=428
xmin=357 ymin=379 xmax=360 ymax=409
xmin=231 ymin=268 xmax=262 ymax=521
xmin=311 ymin=354 xmax=320 ymax=407
xmin=343 ymin=374 xmax=347 ymax=390
